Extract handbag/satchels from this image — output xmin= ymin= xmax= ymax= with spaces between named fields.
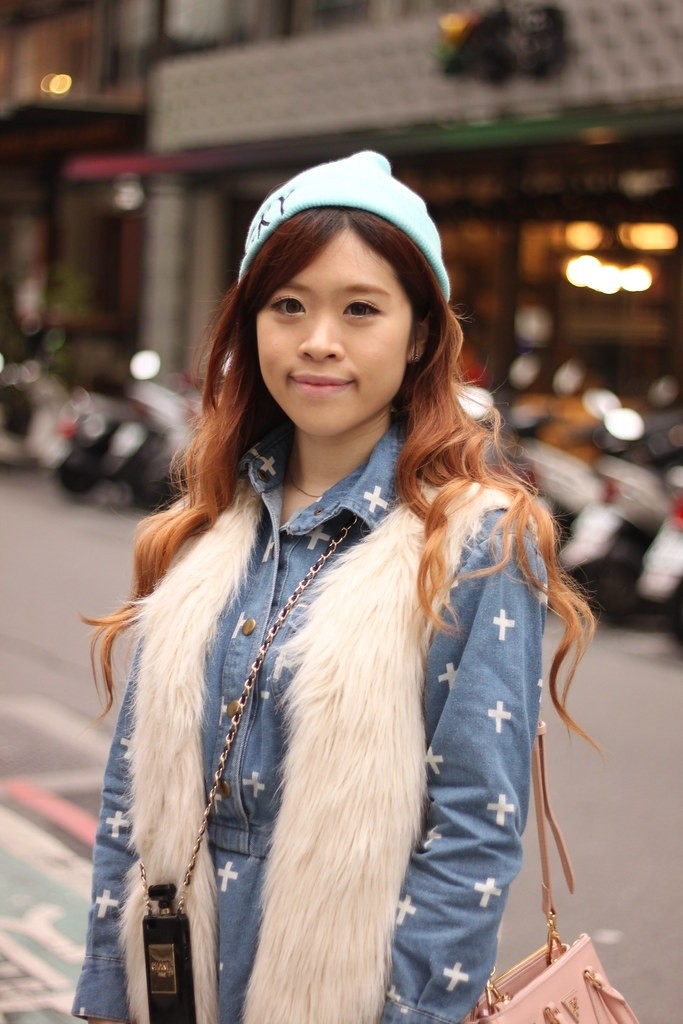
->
xmin=470 ymin=933 xmax=638 ymax=1024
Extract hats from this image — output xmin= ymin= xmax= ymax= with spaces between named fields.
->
xmin=239 ymin=151 xmax=451 ymax=304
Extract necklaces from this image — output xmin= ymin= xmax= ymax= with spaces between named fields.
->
xmin=287 ymin=467 xmax=322 ymax=498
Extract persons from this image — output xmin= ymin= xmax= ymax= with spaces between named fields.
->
xmin=70 ymin=152 xmax=598 ymax=1023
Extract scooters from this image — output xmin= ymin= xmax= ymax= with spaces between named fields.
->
xmin=0 ymin=320 xmax=232 ymax=510
xmin=461 ymin=378 xmax=683 ymax=650
